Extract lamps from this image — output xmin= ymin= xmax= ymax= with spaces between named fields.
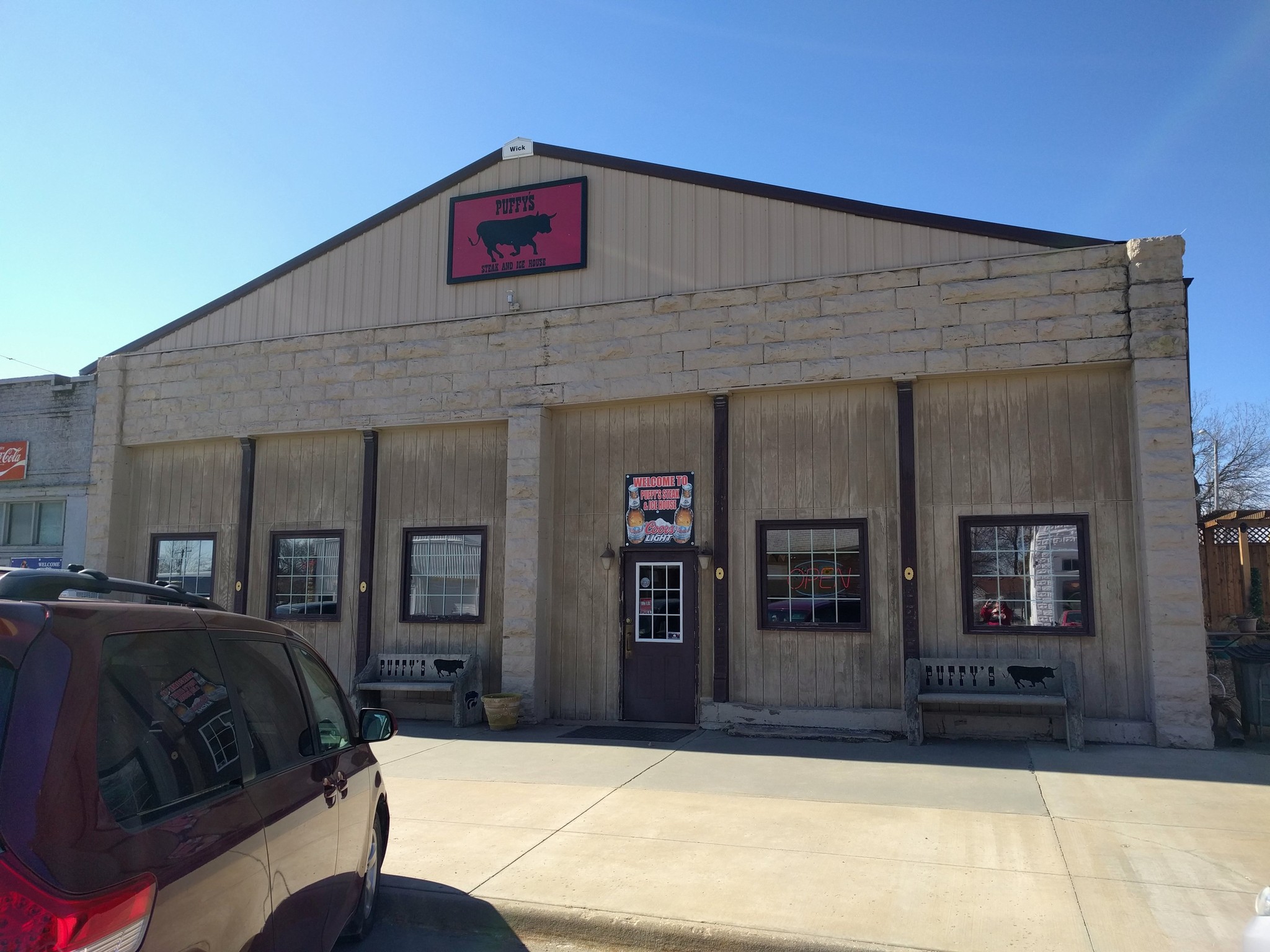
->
xmin=600 ymin=542 xmax=615 ymax=570
xmin=697 ymin=541 xmax=713 ymax=569
xmin=505 ymin=290 xmax=520 ymax=312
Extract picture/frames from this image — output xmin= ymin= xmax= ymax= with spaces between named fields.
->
xmin=447 ymin=176 xmax=588 ymax=285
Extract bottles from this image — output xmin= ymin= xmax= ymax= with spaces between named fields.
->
xmin=163 ymin=695 xmax=195 ymax=723
xmin=626 ymin=484 xmax=645 ymax=544
xmin=673 ymin=483 xmax=693 ymax=544
xmin=193 ymin=672 xmax=216 ymax=694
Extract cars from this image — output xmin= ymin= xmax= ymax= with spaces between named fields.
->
xmin=768 ymin=596 xmax=862 ymax=625
xmin=275 ymin=601 xmax=336 ymax=615
xmin=1054 ymin=610 xmax=1082 ymax=629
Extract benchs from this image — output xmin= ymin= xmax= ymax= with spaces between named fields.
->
xmin=904 ymin=657 xmax=1085 ymax=753
xmin=350 ymin=653 xmax=484 ymax=728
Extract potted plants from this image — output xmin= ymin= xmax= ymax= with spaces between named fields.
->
xmin=1236 ymin=614 xmax=1259 ymax=633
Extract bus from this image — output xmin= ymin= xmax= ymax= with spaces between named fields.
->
xmin=156 ymin=571 xmax=211 ymax=600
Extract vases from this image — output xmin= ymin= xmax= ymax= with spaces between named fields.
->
xmin=480 ymin=693 xmax=523 ymax=731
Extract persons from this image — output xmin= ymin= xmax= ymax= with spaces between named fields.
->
xmin=20 ymin=561 xmax=29 ymax=569
xmin=981 ymin=596 xmax=1011 ymax=627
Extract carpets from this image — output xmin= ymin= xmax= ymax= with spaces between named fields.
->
xmin=555 ymin=726 xmax=697 ymax=743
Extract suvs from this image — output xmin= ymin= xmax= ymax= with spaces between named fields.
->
xmin=0 ymin=562 xmax=400 ymax=952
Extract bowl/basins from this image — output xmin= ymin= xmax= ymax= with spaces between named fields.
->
xmin=481 ymin=692 xmax=523 ymax=732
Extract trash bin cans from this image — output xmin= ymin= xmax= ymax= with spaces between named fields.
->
xmin=1224 ymin=644 xmax=1270 ymax=738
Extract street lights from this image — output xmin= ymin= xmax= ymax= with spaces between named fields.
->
xmin=1198 ymin=429 xmax=1220 ymax=513
